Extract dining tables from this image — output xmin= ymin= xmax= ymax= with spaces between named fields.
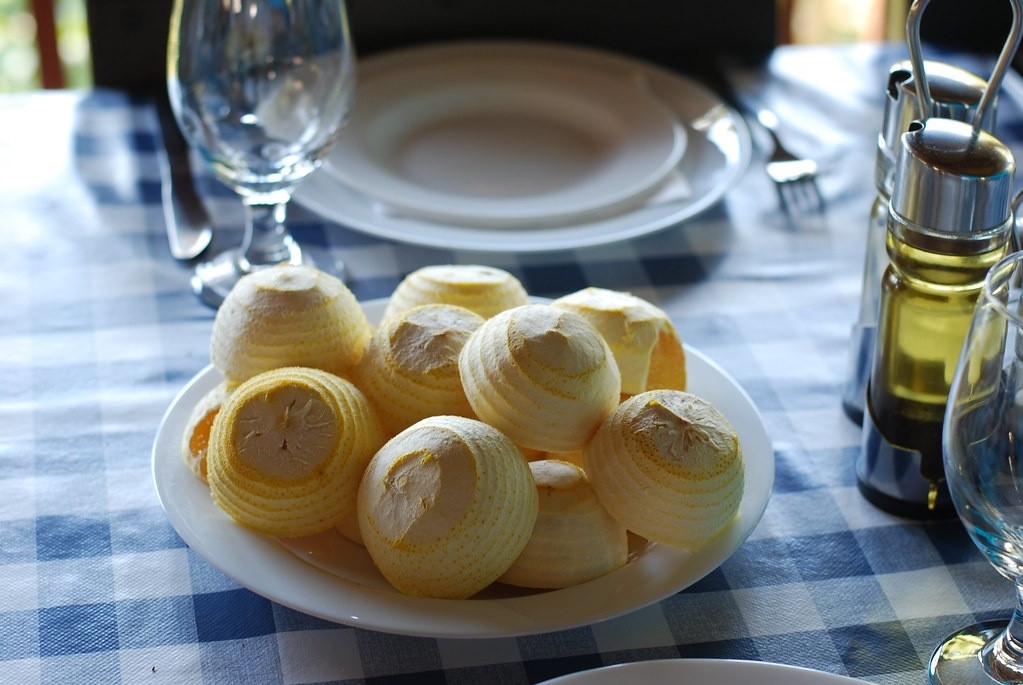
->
xmin=2 ymin=39 xmax=1023 ymax=684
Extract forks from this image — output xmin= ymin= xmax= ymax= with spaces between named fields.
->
xmin=683 ymin=46 xmax=827 ymax=218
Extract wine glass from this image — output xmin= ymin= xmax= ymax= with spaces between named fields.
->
xmin=166 ymin=0 xmax=355 ymax=308
xmin=926 ymin=251 xmax=1022 ymax=685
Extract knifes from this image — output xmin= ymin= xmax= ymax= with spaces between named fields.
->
xmin=152 ymin=97 xmax=211 ymax=260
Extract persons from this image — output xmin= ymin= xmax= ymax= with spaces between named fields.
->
xmin=83 ymin=0 xmax=774 ymax=96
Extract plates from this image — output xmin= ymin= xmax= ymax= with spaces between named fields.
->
xmin=152 ymin=294 xmax=775 ymax=642
xmin=321 ymin=58 xmax=690 ymax=225
xmin=293 ymin=41 xmax=752 ymax=256
xmin=534 ymin=659 xmax=875 ymax=685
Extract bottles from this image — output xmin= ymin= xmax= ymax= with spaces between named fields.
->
xmin=841 ymin=60 xmax=1000 ymax=428
xmin=854 ymin=117 xmax=1017 ymax=520
